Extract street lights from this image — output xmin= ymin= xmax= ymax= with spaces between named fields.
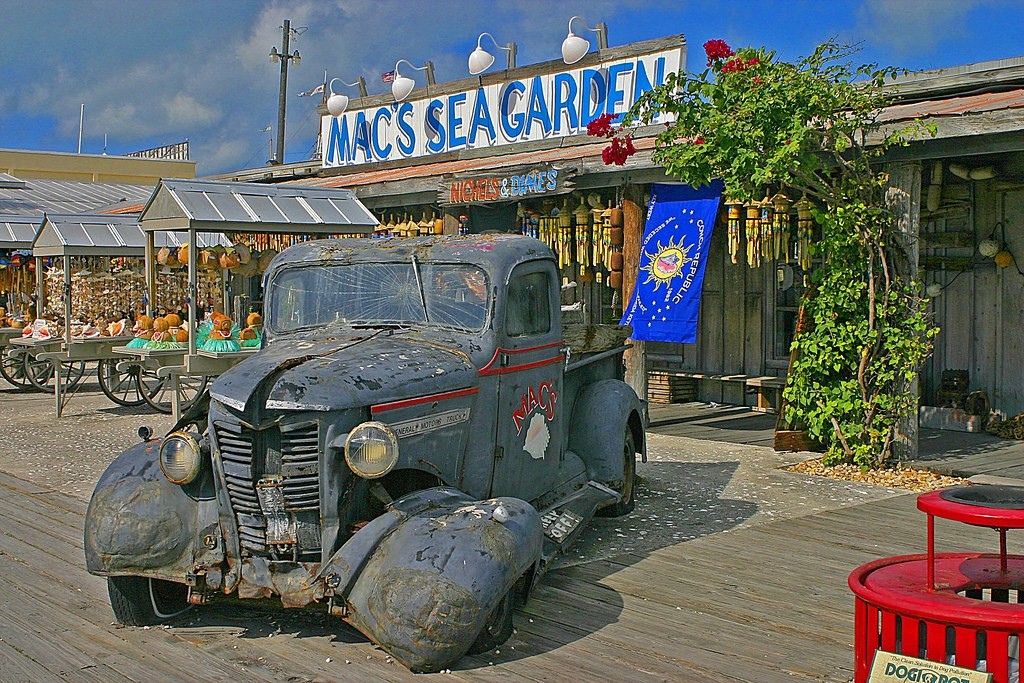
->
xmin=269 ymin=47 xmax=301 ymax=164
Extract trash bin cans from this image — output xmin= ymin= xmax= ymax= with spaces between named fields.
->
xmin=848 ymin=484 xmax=1024 ymax=683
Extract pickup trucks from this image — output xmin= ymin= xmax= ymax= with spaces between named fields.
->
xmin=85 ymin=230 xmax=651 ymax=674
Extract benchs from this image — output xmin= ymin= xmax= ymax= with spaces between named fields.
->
xmin=646 ymin=370 xmax=785 ymax=415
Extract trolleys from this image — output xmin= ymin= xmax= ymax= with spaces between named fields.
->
xmin=0 ymin=178 xmax=383 ymax=422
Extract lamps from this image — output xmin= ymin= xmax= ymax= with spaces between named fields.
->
xmin=562 ymin=16 xmax=603 ymax=64
xmin=392 ymin=59 xmax=429 ymax=102
xmin=467 ymin=33 xmax=507 ymax=74
xmin=327 ymin=78 xmax=361 ymax=117
